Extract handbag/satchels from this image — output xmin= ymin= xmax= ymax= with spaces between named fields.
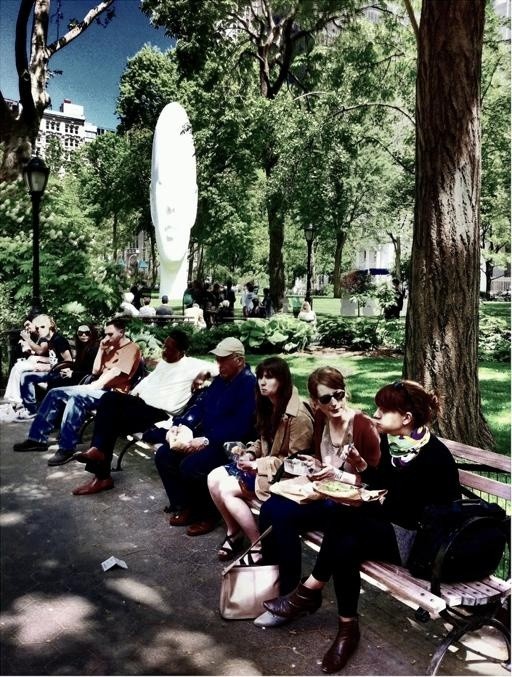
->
xmin=408 ymin=484 xmax=510 ymax=597
xmin=220 ymin=526 xmax=282 ymax=622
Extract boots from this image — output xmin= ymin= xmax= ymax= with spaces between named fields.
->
xmin=262 ymin=574 xmax=322 ymax=619
xmin=322 ymin=615 xmax=363 ymax=674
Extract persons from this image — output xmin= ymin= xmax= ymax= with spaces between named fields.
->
xmin=252 ymin=366 xmax=381 ymax=628
xmin=263 ymin=381 xmax=462 ymax=674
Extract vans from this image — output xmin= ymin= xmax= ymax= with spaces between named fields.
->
xmin=359 ymin=266 xmax=392 ymax=289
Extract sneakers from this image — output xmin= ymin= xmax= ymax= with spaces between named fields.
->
xmin=0 ymin=403 xmax=38 ymax=424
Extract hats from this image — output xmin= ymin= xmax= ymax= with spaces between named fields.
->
xmin=207 ymin=336 xmax=246 ymax=358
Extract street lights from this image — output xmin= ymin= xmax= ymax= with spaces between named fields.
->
xmin=20 ymin=146 xmax=56 ymax=311
xmin=127 ymin=253 xmax=138 ymax=267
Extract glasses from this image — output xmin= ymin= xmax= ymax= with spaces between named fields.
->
xmin=78 ymin=330 xmax=90 ymax=336
xmin=25 ymin=322 xmax=46 ymax=330
xmin=313 ymin=391 xmax=345 ymax=405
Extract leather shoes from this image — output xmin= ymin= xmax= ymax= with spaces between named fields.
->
xmin=253 ymin=608 xmax=286 ymax=627
xmin=74 ymin=446 xmax=107 ymax=467
xmin=46 ymin=448 xmax=77 ymax=467
xmin=169 ymin=509 xmax=196 ymax=527
xmin=13 ymin=440 xmax=48 ymax=453
xmin=186 ymin=519 xmax=220 ymax=536
xmin=73 ymin=476 xmax=115 ymax=496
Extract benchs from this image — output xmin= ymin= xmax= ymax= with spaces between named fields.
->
xmin=36 ymin=342 xmax=212 ymax=471
xmin=246 ymin=416 xmax=510 ymax=675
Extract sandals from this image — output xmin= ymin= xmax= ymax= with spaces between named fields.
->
xmin=218 ymin=531 xmax=240 ymax=563
xmin=233 ymin=546 xmax=264 ymax=567
